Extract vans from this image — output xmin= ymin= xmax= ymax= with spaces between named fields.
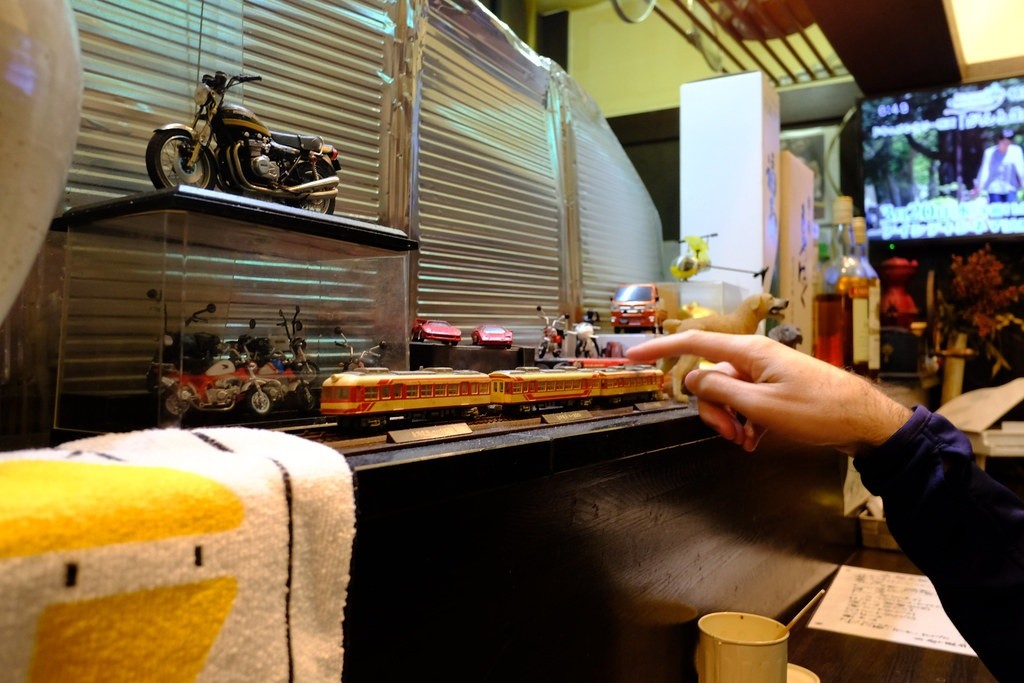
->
xmin=609 ymin=283 xmax=666 ymax=334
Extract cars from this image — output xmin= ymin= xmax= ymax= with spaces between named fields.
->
xmin=411 ymin=317 xmax=462 ymax=346
xmin=471 ymin=324 xmax=514 ymax=349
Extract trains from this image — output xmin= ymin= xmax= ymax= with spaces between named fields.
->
xmin=320 ymin=363 xmax=665 ymax=426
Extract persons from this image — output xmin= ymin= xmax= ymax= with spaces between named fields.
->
xmin=626 ymin=330 xmax=1024 ymax=683
xmin=974 ymin=132 xmax=1024 ymax=204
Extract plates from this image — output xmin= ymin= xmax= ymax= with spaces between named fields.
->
xmin=787 ymin=663 xmax=821 ymax=683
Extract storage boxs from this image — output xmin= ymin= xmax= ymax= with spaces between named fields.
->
xmin=50 ymin=187 xmax=419 ymax=435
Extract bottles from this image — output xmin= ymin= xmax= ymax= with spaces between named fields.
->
xmin=815 ymin=195 xmax=871 ymax=377
xmin=811 ymin=242 xmax=829 ymax=358
xmin=852 ymin=216 xmax=881 ymax=382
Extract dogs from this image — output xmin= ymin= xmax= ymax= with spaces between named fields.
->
xmin=765 ymin=322 xmax=804 ymax=350
xmin=651 ymin=290 xmax=790 ymax=404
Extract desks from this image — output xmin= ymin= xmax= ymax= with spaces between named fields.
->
xmin=783 ymin=544 xmax=993 ymax=683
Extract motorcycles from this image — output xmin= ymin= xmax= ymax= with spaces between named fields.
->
xmin=536 ymin=306 xmax=600 ymax=358
xmin=145 ymin=69 xmax=341 ymax=215
xmin=145 ymin=289 xmax=389 ymax=419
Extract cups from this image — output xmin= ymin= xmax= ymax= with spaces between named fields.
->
xmin=697 ymin=611 xmax=789 ymax=683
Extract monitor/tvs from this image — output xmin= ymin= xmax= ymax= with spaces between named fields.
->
xmin=854 ymin=75 xmax=1024 ymax=251
